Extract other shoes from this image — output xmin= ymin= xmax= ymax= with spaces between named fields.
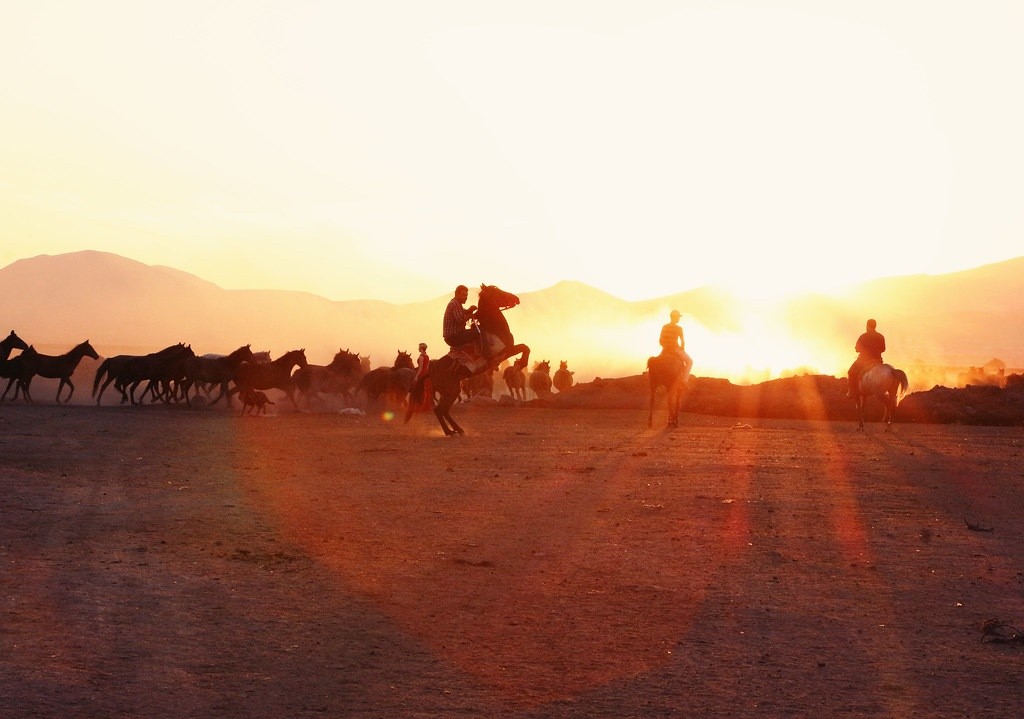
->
xmin=682 ymin=382 xmax=691 ymax=391
xmin=846 ymin=389 xmax=857 ymax=399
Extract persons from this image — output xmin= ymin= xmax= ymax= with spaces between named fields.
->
xmin=442 ymin=285 xmax=499 ymax=371
xmin=845 ymin=319 xmax=886 ymax=400
xmin=659 ymin=310 xmax=693 ymax=392
xmin=411 ymin=343 xmax=430 ymax=385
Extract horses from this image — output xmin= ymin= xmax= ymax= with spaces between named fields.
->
xmin=404 ymin=283 xmax=531 ymax=438
xmin=647 ymin=333 xmax=684 ymax=428
xmin=853 ymin=363 xmax=910 ymax=432
xmin=0 ymin=331 xmax=28 ymax=371
xmin=3 ymin=338 xmax=577 ymax=420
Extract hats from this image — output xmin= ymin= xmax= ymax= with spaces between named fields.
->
xmin=670 ymin=310 xmax=683 ymax=317
